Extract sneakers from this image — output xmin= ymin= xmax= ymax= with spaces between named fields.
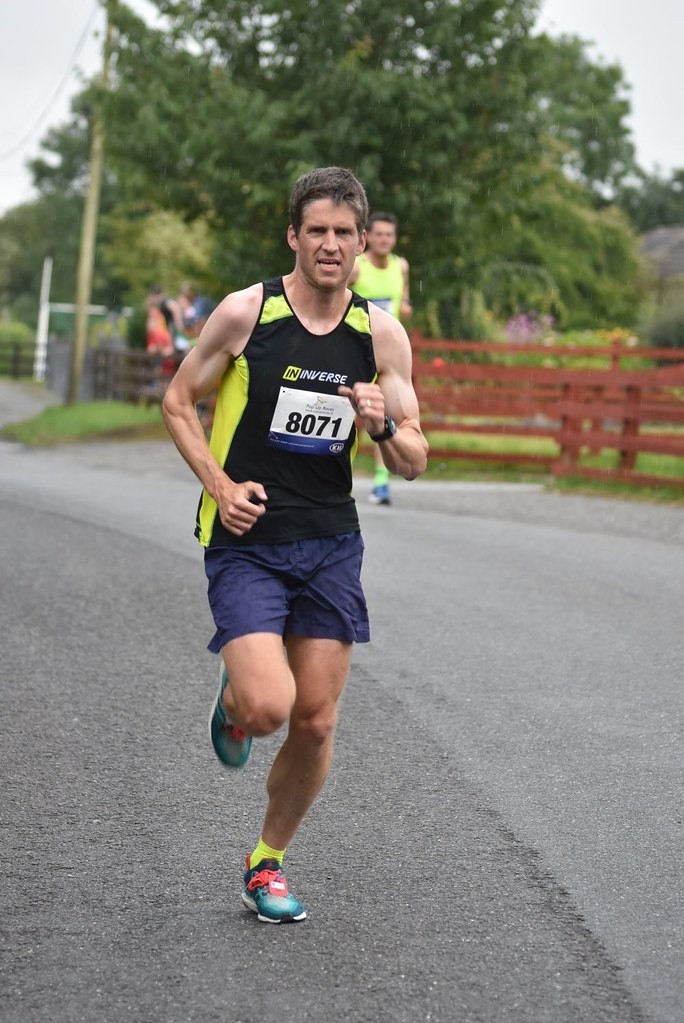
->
xmin=240 ymin=853 xmax=306 ymax=926
xmin=210 ymin=660 xmax=250 ymax=767
xmin=369 ymin=485 xmax=388 ymax=505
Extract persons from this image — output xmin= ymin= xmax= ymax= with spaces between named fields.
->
xmin=143 ymin=163 xmax=431 ymax=930
xmin=144 ymin=281 xmax=219 ymax=430
xmin=335 ymin=210 xmax=412 ymax=509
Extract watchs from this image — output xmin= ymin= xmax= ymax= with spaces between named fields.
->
xmin=368 ymin=414 xmax=399 ymax=442
